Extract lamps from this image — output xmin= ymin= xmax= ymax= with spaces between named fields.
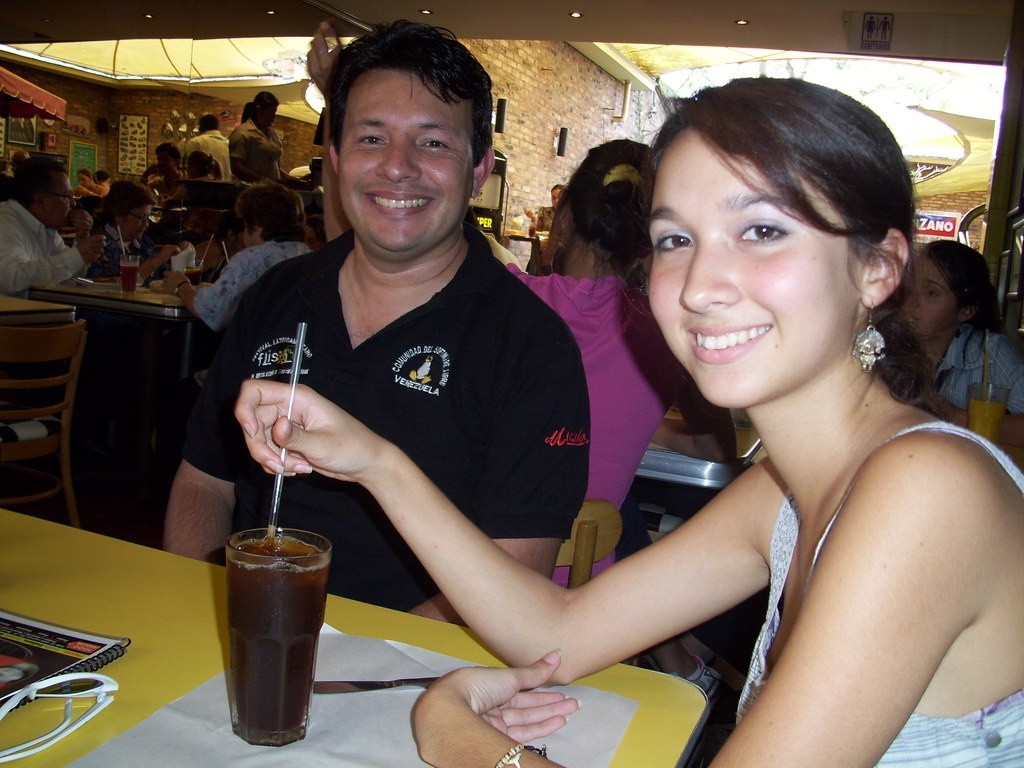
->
xmin=553 ymin=128 xmax=568 ymax=157
xmin=490 ymin=98 xmax=507 ymax=133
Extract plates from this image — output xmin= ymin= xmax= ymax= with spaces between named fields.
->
xmin=73 ymin=277 xmax=142 ymax=289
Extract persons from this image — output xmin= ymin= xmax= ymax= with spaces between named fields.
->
xmin=235 ymin=76 xmax=1024 ymax=768
xmin=683 ymin=241 xmax=1024 ymax=723
xmin=523 ymin=184 xmax=564 ymax=231
xmin=306 ymin=19 xmax=738 ymax=699
xmin=0 ymin=91 xmax=319 ymax=503
xmin=160 ymin=18 xmax=591 ymax=628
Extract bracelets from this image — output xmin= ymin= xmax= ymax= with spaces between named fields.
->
xmin=174 ymin=281 xmax=190 ymax=297
xmin=495 ymin=744 xmax=542 ymax=768
xmin=146 ymin=260 xmax=155 ymax=277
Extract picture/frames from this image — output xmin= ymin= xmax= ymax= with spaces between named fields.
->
xmin=5 ymin=114 xmax=38 ymax=147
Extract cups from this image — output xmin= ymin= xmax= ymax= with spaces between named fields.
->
xmin=120 ymin=254 xmax=140 ymax=295
xmin=967 ymin=382 xmax=1011 ymax=445
xmin=186 ymin=265 xmax=203 ymax=285
xmin=226 ymin=527 xmax=332 ymax=747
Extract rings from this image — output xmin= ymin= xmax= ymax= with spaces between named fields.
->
xmin=87 ymin=225 xmax=90 ymax=231
xmin=317 ymin=49 xmax=327 ymax=57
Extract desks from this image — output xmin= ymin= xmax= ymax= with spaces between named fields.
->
xmin=503 ymin=234 xmax=536 ymax=243
xmin=29 ymin=280 xmax=202 ymax=466
xmin=0 ymin=509 xmax=710 ymax=768
xmin=931 ymin=402 xmax=1024 ymax=476
xmin=635 ymin=406 xmax=764 ymax=489
xmin=0 ymin=294 xmax=76 ymax=325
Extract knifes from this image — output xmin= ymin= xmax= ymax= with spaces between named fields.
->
xmin=313 ymin=677 xmax=443 ymax=694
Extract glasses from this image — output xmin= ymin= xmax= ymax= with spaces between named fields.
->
xmin=128 ymin=212 xmax=151 ymax=224
xmin=47 ymin=191 xmax=72 ymax=200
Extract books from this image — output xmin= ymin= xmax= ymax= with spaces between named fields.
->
xmin=0 ymin=608 xmax=131 ymax=712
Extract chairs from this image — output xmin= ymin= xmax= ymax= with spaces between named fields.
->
xmin=555 ymin=500 xmax=623 ymax=590
xmin=534 ymin=234 xmax=551 ymax=276
xmin=0 ymin=319 xmax=87 ymax=531
